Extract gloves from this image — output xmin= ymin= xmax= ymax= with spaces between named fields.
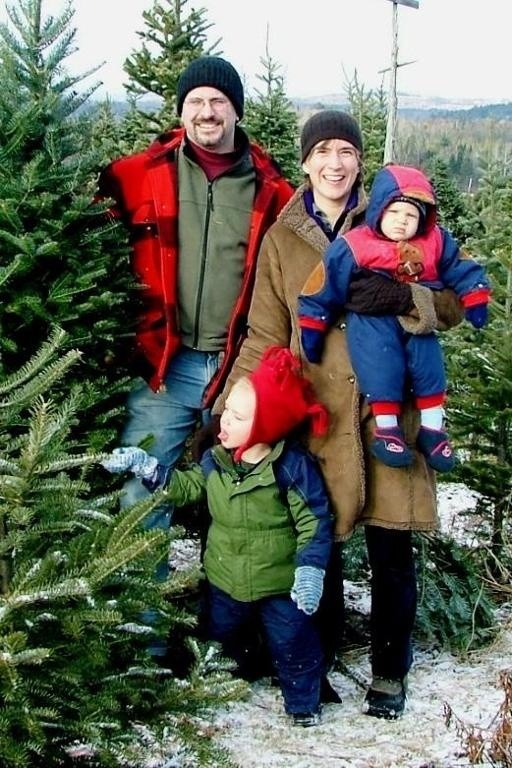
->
xmin=102 ymin=447 xmax=156 ymax=480
xmin=467 ymin=305 xmax=487 ymax=325
xmin=346 ymin=268 xmax=415 ymax=317
xmin=290 ymin=565 xmax=326 ymax=613
xmin=302 ymin=328 xmax=324 ymax=361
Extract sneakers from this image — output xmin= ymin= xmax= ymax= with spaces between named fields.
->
xmin=366 ymin=428 xmax=412 ymax=469
xmin=276 ymin=676 xmax=341 ymax=728
xmin=415 ymin=423 xmax=453 ymax=472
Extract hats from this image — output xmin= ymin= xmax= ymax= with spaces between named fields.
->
xmin=300 ymin=110 xmax=362 ymax=162
xmin=392 ymin=194 xmax=426 ymax=216
xmin=233 ymin=347 xmax=328 ymax=461
xmin=176 ymin=57 xmax=244 ymax=121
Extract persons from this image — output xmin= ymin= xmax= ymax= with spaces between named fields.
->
xmin=193 ymin=111 xmax=437 ymax=720
xmin=295 ymin=163 xmax=493 ymax=475
xmin=99 ymin=347 xmax=335 ymax=729
xmin=83 ymin=51 xmax=294 ymax=695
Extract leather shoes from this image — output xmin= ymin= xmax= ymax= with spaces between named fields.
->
xmin=365 ymin=677 xmax=407 ymax=718
